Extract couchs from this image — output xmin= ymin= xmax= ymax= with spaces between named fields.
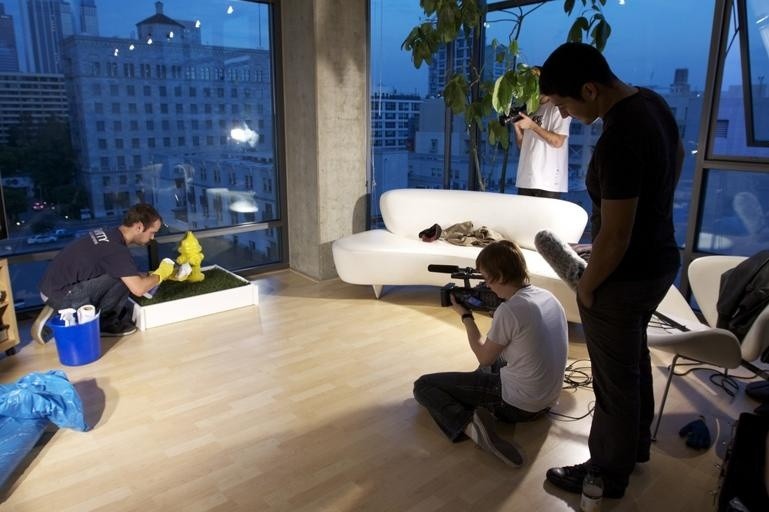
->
xmin=331 ymin=188 xmax=588 ymax=325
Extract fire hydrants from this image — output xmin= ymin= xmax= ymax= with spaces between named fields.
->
xmin=175 ymin=232 xmax=206 ymax=283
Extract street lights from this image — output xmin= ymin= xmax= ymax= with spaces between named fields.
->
xmin=758 ymin=74 xmax=764 ymax=95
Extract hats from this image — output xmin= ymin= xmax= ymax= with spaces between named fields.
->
xmin=419 ymin=224 xmax=442 ymax=242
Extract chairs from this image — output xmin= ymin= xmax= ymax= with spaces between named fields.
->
xmin=647 ymin=255 xmax=769 ymax=442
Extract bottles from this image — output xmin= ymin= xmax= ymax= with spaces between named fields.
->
xmin=580 ymin=463 xmax=605 ymax=510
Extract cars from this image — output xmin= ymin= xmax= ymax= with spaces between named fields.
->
xmin=27 ymin=234 xmax=57 ymax=244
xmin=32 ymin=203 xmax=43 ymax=210
xmin=53 ymin=229 xmax=74 ymax=237
xmin=80 ymin=208 xmax=90 ymax=221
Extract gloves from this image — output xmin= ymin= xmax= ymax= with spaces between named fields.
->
xmin=679 ymin=414 xmax=711 ymax=449
xmin=148 ymin=257 xmax=193 ymax=284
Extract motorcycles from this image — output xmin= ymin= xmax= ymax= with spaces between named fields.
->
xmin=51 ymin=202 xmax=55 ymax=211
xmin=15 ymin=221 xmax=22 ymax=231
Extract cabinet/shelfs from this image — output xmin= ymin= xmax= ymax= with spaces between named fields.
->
xmin=0 ymin=257 xmax=21 ymax=353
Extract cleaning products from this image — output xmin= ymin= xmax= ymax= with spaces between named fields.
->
xmin=57 ymin=308 xmax=77 ymax=326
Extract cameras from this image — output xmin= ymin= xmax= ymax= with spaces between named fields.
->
xmin=500 ymin=103 xmax=534 ymax=127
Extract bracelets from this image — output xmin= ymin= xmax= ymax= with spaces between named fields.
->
xmin=461 ymin=312 xmax=475 ymax=324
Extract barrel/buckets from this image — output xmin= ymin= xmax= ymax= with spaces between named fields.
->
xmin=46 ymin=310 xmax=102 ymax=366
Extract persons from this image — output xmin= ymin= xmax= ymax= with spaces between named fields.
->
xmin=412 ymin=238 xmax=571 ymax=469
xmin=513 ymin=66 xmax=573 ymax=199
xmin=37 ymin=202 xmax=194 ymax=339
xmin=538 ymin=40 xmax=690 ymax=501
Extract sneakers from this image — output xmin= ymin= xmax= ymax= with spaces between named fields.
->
xmin=472 ymin=407 xmax=522 ymax=468
xmin=547 ymin=461 xmax=626 ymax=499
xmin=101 ymin=321 xmax=136 ymax=337
xmin=31 ymin=305 xmax=54 ymax=345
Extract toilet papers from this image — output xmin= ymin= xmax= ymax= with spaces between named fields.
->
xmin=76 ymin=304 xmax=101 ymax=325
